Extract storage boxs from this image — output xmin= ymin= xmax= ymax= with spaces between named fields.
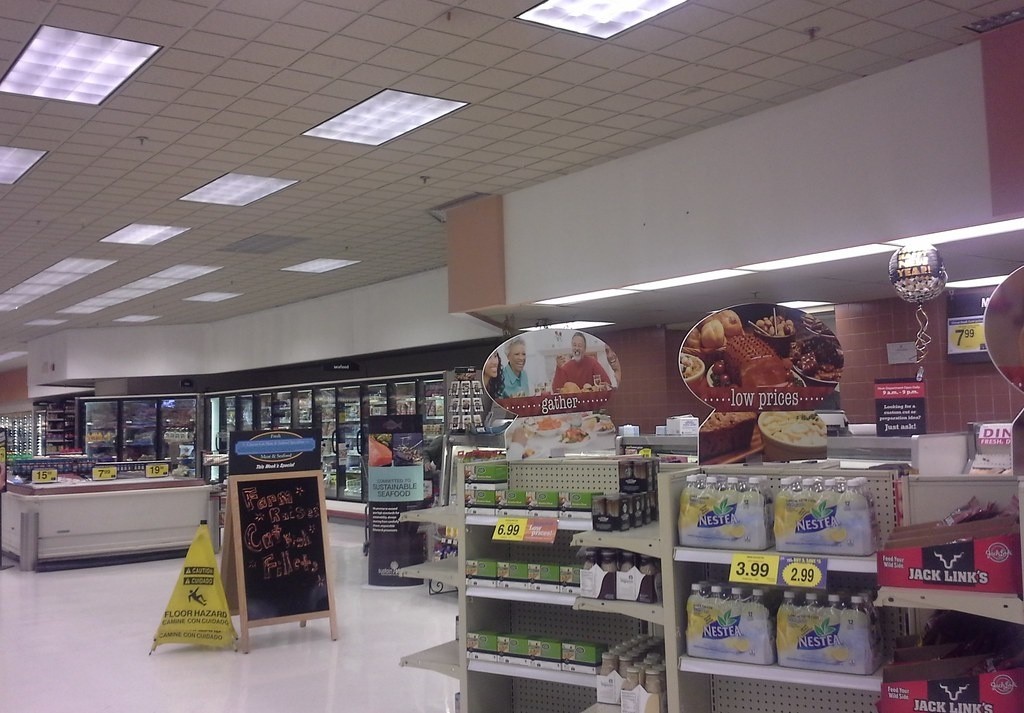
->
xmin=879 ymin=635 xmax=1024 ymax=713
xmin=877 ymin=509 xmax=1021 ymax=591
xmin=656 ymin=414 xmax=699 ymax=436
xmin=619 ymin=426 xmax=640 ymax=438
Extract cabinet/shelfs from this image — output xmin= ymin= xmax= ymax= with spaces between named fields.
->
xmin=396 ymin=456 xmax=604 ymax=713
xmin=566 ymin=468 xmax=711 ymax=713
xmin=875 ymin=434 xmax=1024 ymax=713
xmin=47 ymin=409 xmax=74 ymax=455
xmin=675 ymin=464 xmax=900 ymax=713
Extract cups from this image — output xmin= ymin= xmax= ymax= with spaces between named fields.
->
xmin=594 ymin=374 xmax=601 ymax=389
xmin=533 ymin=383 xmax=546 ymax=397
xmin=753 ymin=327 xmax=796 ymax=357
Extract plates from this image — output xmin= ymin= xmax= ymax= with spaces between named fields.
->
xmin=707 ymin=360 xmax=807 ymax=387
xmin=524 ymin=413 xmax=615 ymax=446
xmin=789 ymin=348 xmax=844 ymax=383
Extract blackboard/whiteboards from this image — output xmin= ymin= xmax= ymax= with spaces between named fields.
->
xmin=220 ymin=469 xmax=336 ymax=629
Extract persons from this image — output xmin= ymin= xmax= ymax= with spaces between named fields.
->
xmin=484 ymin=351 xmax=505 ymax=397
xmin=552 ymin=332 xmax=613 ymax=393
xmin=424 ymin=436 xmax=442 ymax=503
xmin=502 ymin=338 xmax=530 ymax=397
xmin=605 ymin=344 xmax=620 ymax=384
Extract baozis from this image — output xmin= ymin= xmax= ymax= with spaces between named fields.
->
xmin=685 ymin=310 xmax=744 ymax=348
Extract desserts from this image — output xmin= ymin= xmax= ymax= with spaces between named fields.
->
xmin=724 ymin=334 xmax=786 ymax=388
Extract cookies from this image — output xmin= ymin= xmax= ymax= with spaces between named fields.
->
xmin=789 ymin=340 xmax=843 ymax=381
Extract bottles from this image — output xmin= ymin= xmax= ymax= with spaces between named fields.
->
xmin=14 ymin=458 xmax=146 ymax=475
xmin=679 ymin=473 xmax=884 ymax=675
xmin=582 ymin=550 xmax=666 ymax=713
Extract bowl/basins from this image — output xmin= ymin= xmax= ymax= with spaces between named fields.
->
xmin=680 ymin=353 xmax=705 ymax=381
xmin=758 ymin=412 xmax=827 ymax=462
